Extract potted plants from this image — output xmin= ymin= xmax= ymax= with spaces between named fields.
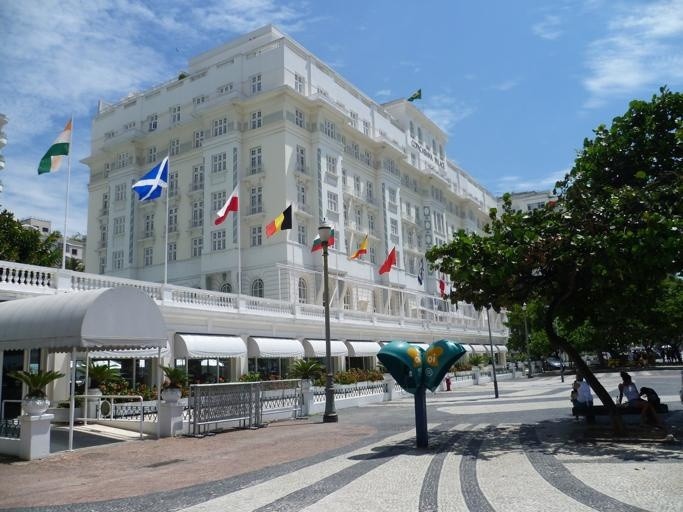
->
xmin=468 ymin=353 xmax=491 ymax=371
xmin=6 ymin=365 xmax=64 ymax=415
xmin=73 ymin=359 xmax=121 ymax=401
xmin=377 ymin=360 xmax=393 ymax=380
xmin=158 ymin=362 xmax=192 ymax=404
xmin=286 ymin=357 xmax=325 ymax=389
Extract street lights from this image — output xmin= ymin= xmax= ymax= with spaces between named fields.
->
xmin=521 ymin=301 xmax=535 ymax=379
xmin=317 ymin=216 xmax=339 ymax=422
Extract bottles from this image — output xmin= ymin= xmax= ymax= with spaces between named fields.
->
xmin=616 ymin=396 xmax=621 ymax=406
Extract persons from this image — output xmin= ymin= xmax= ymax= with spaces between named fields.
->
xmin=570 ymin=372 xmax=594 ymax=420
xmin=618 ymin=371 xmax=664 ymax=427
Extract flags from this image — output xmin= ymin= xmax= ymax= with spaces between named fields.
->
xmin=263 ymin=205 xmax=291 ymax=239
xmin=346 ymin=234 xmax=368 ymax=259
xmin=310 ymin=225 xmax=334 ymax=254
xmin=378 ymin=246 xmax=395 ymax=274
xmin=407 ymin=89 xmax=422 ymax=102
xmin=415 ymin=259 xmax=424 ymax=284
xmin=35 ymin=117 xmax=74 ymax=175
xmin=211 ymin=183 xmax=239 ymax=225
xmin=439 ymin=274 xmax=445 ymax=298
xmin=130 ymin=156 xmax=168 ymax=201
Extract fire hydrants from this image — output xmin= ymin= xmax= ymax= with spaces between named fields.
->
xmin=444 ymin=375 xmax=453 ymax=393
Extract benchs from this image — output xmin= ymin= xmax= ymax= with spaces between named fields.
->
xmin=571 ymin=396 xmax=670 ymax=422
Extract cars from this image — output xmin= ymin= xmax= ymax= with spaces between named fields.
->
xmin=544 ymin=344 xmax=683 ymax=372
